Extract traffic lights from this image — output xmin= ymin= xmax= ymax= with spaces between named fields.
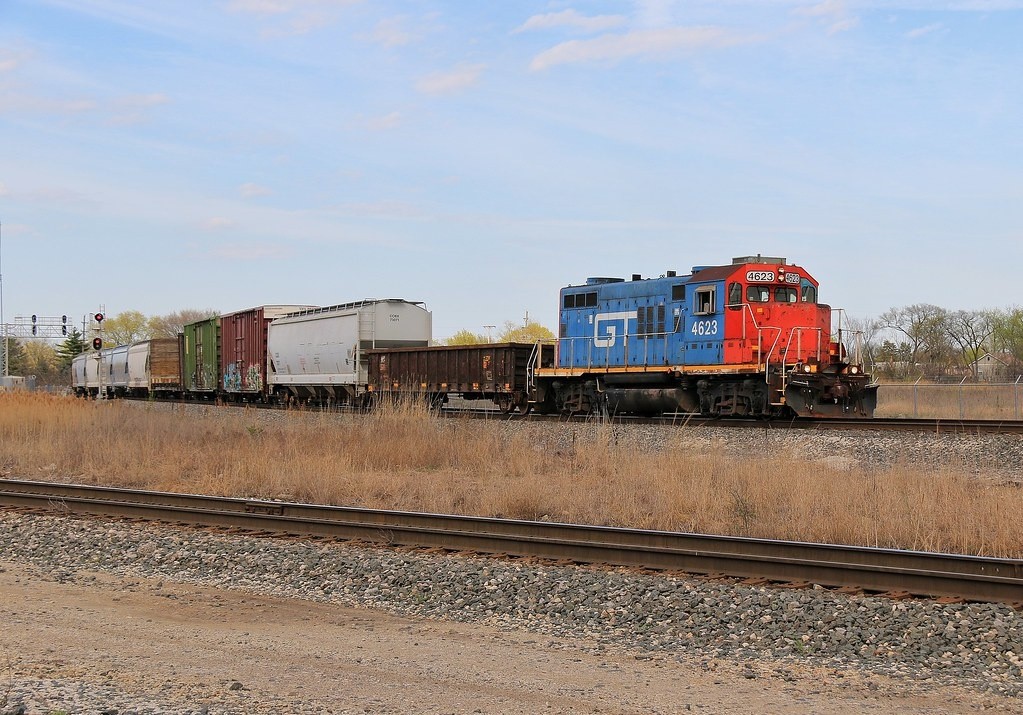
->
xmin=93 ymin=338 xmax=102 ymax=350
xmin=95 ymin=314 xmax=103 ymax=322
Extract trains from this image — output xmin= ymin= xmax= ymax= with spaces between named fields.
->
xmin=69 ymin=254 xmax=881 ymax=421
